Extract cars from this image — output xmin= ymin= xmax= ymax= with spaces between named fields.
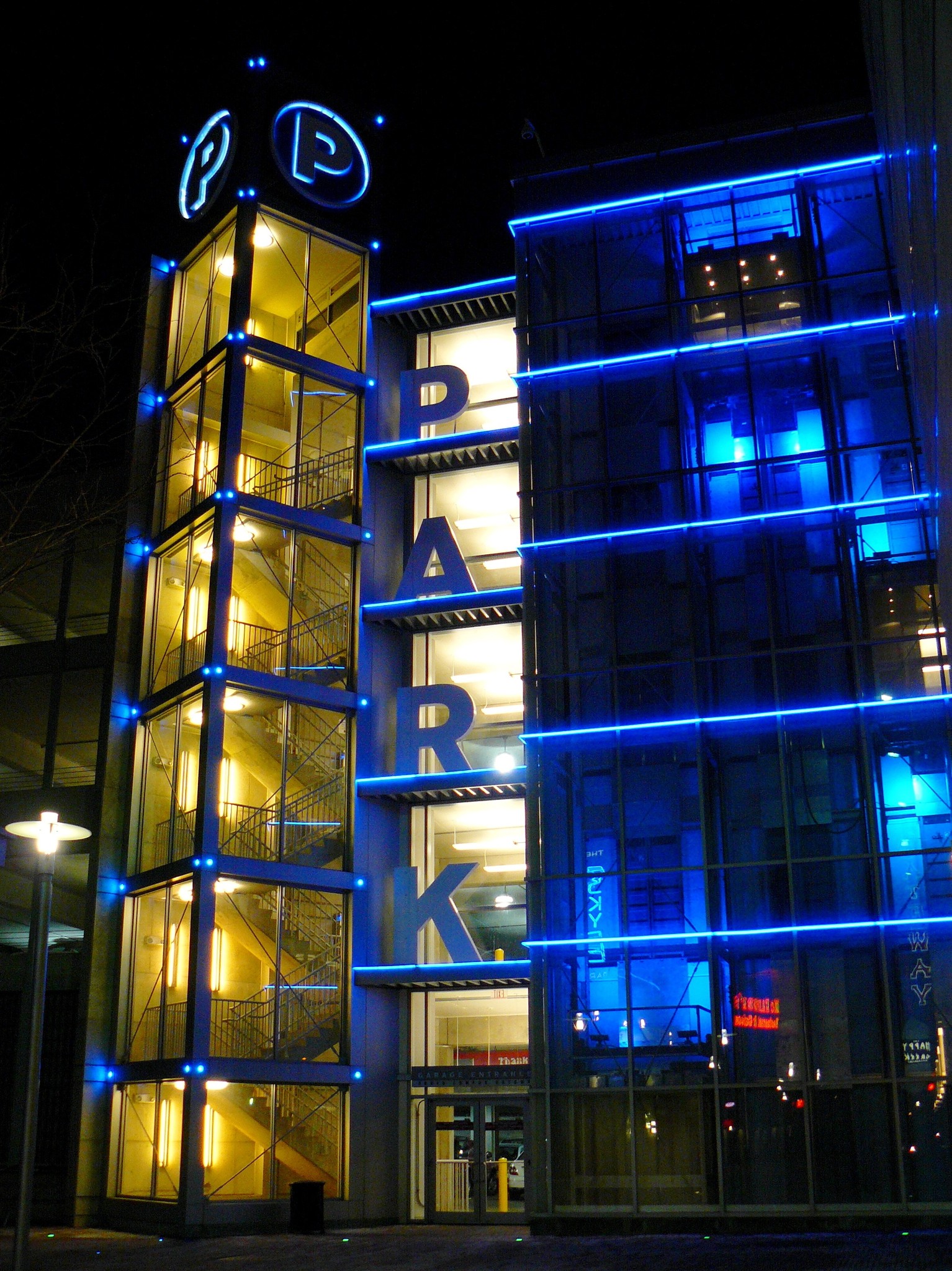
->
xmin=507 ymin=1151 xmax=524 ymax=1199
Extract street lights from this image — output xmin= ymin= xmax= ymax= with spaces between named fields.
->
xmin=1 ymin=807 xmax=94 ymax=1268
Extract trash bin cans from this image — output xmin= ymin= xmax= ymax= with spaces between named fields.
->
xmin=289 ymin=1180 xmax=326 ymax=1234
